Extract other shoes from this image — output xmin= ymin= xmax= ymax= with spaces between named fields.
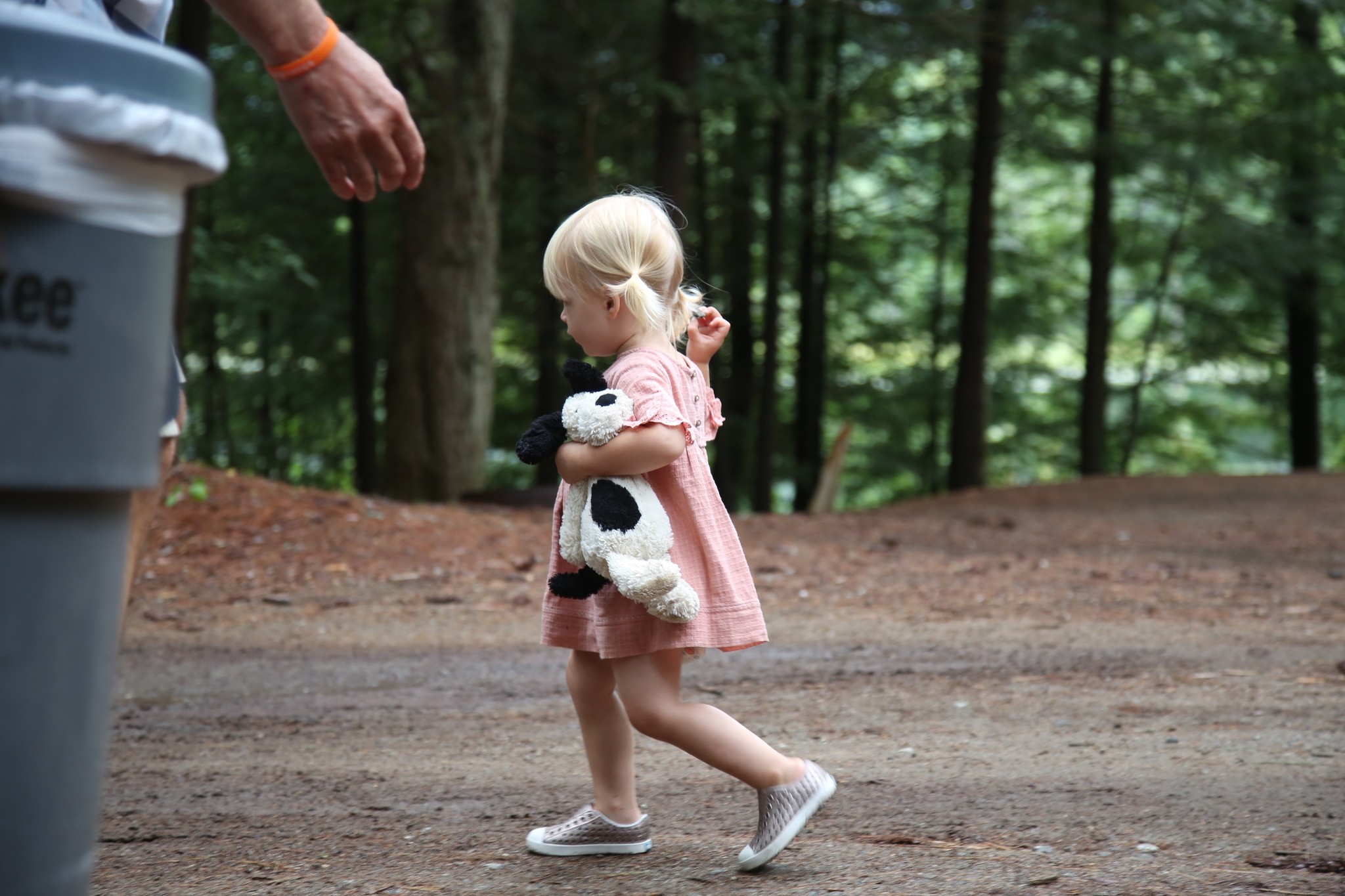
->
xmin=526 ymin=805 xmax=652 ymax=856
xmin=737 ymin=758 xmax=836 ymax=871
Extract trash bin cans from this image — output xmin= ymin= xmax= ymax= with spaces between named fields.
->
xmin=0 ymin=0 xmax=226 ymax=896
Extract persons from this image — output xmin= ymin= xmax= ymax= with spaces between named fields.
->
xmin=526 ymin=193 xmax=838 ymax=871
xmin=0 ymin=0 xmax=427 ymax=624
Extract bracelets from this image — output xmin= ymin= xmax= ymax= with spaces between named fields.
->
xmin=264 ymin=18 xmax=340 ymax=82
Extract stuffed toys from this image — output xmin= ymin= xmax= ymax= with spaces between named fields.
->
xmin=515 ymin=358 xmax=702 ymax=624
